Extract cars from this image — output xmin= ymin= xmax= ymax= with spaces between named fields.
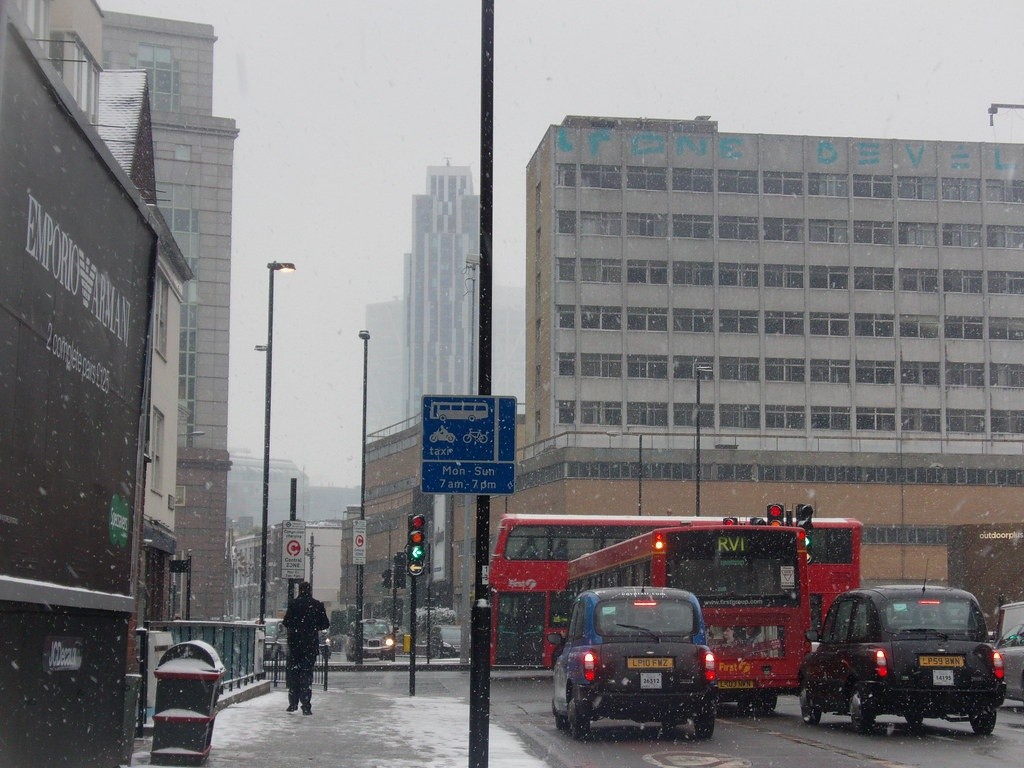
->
xmin=251 ymin=618 xmax=290 ymax=659
xmin=428 ymin=626 xmax=472 ymax=659
xmin=345 ymin=620 xmax=395 ymax=662
xmin=545 ymin=585 xmax=718 ymax=742
xmin=994 ymin=622 xmax=1024 ymax=708
xmin=796 ymin=585 xmax=1006 ymax=736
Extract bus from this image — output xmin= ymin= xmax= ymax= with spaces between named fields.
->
xmin=564 ymin=524 xmax=810 ymax=713
xmin=491 ymin=514 xmax=864 ymax=669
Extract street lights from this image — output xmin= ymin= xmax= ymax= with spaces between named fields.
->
xmin=256 ymin=260 xmax=296 ymax=629
xmin=350 ymin=329 xmax=371 ymax=663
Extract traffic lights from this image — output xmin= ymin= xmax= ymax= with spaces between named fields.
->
xmin=767 ymin=504 xmax=784 ymax=528
xmin=796 ymin=504 xmax=815 ymax=565
xmin=723 ymin=517 xmax=738 ymax=525
xmin=407 ymin=513 xmax=426 ymax=577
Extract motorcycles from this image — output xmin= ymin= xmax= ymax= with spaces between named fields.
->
xmin=320 ymin=638 xmax=332 ymax=659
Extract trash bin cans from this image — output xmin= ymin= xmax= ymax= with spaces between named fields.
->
xmin=147 ymin=641 xmax=228 ymax=767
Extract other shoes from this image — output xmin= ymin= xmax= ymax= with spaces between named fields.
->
xmin=287 ymin=705 xmax=297 ymax=712
xmin=303 ymin=710 xmax=312 ymax=716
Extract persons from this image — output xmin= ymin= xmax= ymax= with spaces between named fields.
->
xmin=282 ymin=581 xmax=331 ymax=716
